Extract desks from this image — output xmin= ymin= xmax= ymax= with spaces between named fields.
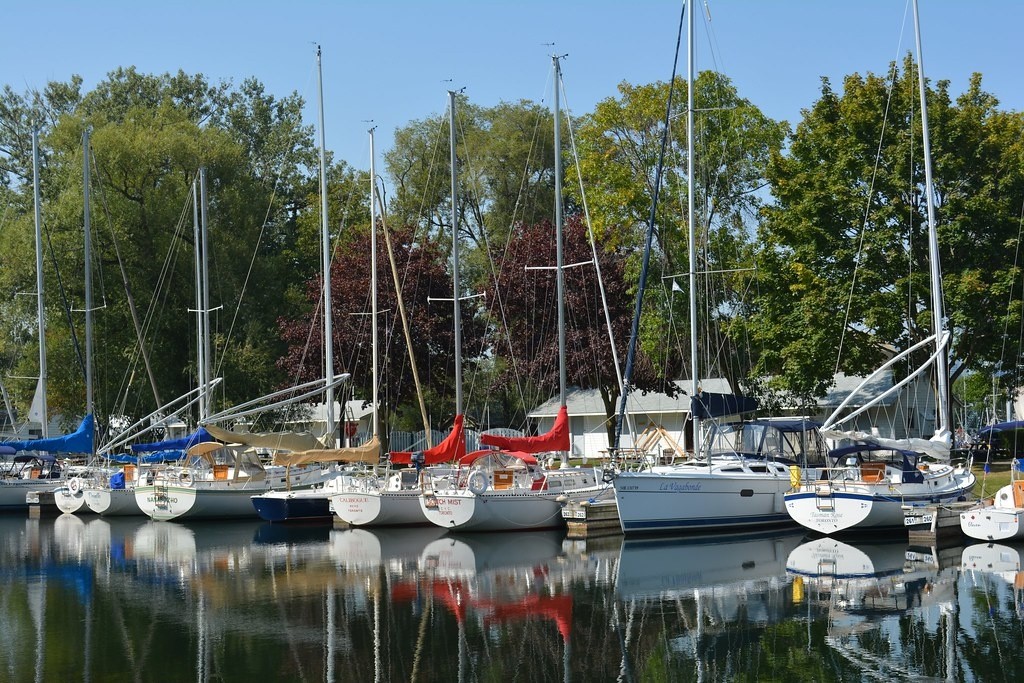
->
xmin=599 ymin=450 xmax=641 ymax=463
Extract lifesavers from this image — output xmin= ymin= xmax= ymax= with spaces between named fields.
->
xmin=68 ymin=476 xmax=80 ymax=495
xmin=467 ymin=471 xmax=488 ymax=496
xmin=178 ymin=469 xmax=195 ymax=488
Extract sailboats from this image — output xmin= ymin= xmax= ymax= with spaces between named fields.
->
xmin=792 ymin=1 xmax=979 ymax=538
xmin=601 ymin=1 xmax=834 ymax=538
xmin=416 ymin=40 xmax=650 ymax=533
xmin=27 ymin=499 xmax=1024 ymax=682
xmin=0 ymin=43 xmax=535 ymax=531
xmin=957 ymin=210 xmax=1024 ymax=543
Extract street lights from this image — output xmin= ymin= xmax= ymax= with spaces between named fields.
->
xmin=985 ymin=393 xmax=1002 ymax=426
